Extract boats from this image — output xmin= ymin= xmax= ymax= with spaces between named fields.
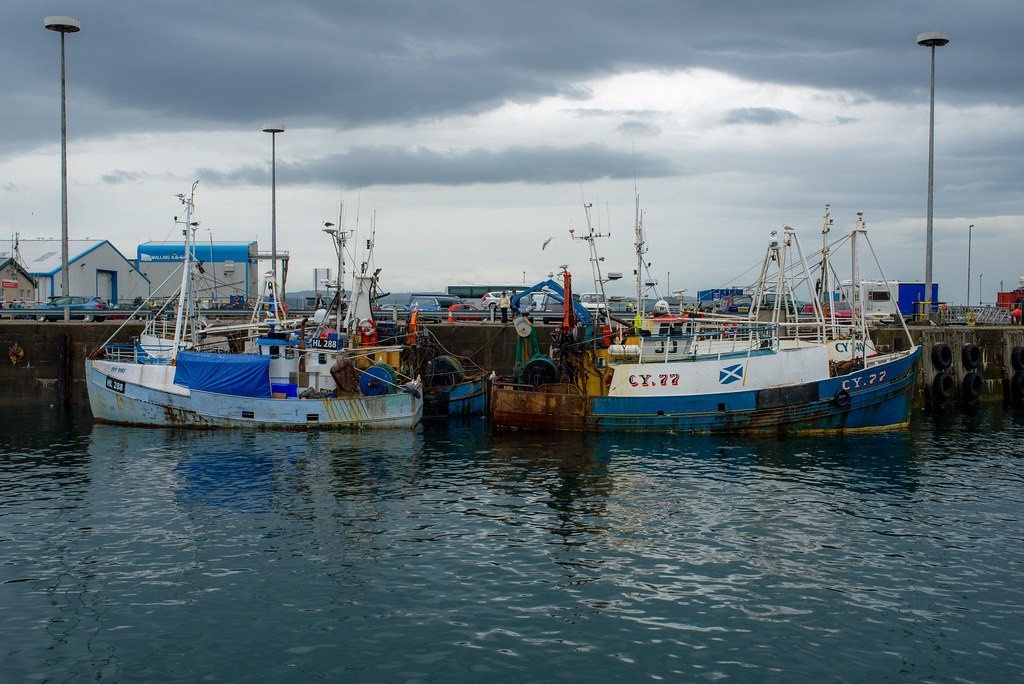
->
xmin=491 ymin=141 xmax=922 ymax=435
xmin=84 ymin=180 xmax=424 ymax=430
xmin=416 ymin=365 xmax=488 ymax=418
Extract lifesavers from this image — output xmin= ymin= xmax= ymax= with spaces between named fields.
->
xmin=358 ymin=318 xmax=377 ymax=336
xmin=234 ymin=296 xmax=239 ymax=301
xmin=720 ymin=319 xmax=737 ymax=338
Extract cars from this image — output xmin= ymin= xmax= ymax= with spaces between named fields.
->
xmin=373 ymin=277 xmax=1024 ymax=326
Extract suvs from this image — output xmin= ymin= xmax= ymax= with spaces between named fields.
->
xmin=29 ymin=296 xmax=109 ymax=321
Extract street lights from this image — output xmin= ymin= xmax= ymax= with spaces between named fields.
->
xmin=979 ymin=273 xmax=983 ymax=307
xmin=966 ymin=225 xmax=973 ymax=323
xmin=44 ymin=16 xmax=82 ymax=296
xmin=914 ymin=31 xmax=951 ymax=325
xmin=262 ymin=123 xmax=285 ymax=299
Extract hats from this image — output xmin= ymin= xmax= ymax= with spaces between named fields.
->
xmin=502 ymin=292 xmax=506 ymax=294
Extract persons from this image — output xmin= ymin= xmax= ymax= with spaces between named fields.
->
xmin=315 ymin=293 xmax=324 ymax=309
xmin=682 ymin=303 xmax=722 ymax=320
xmin=0 ymin=297 xmax=28 ymax=321
xmin=510 ymin=289 xmax=520 ymax=324
xmin=497 ymin=292 xmax=511 ymax=324
